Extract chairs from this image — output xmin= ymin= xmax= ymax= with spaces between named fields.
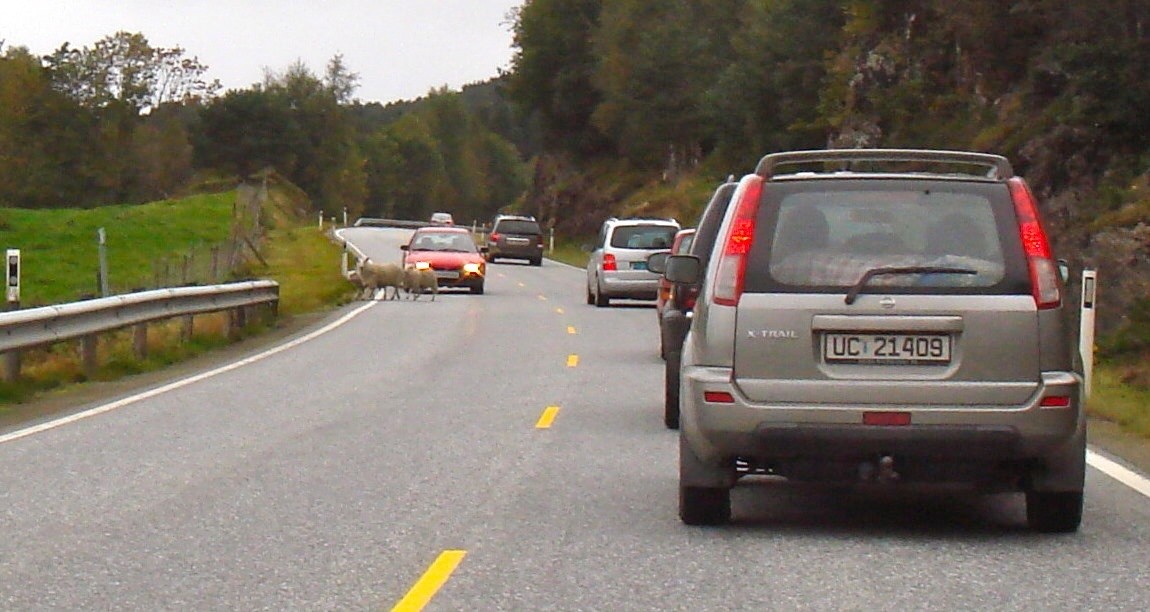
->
xmin=651 ymin=237 xmax=667 ymax=248
xmin=923 ymin=214 xmax=989 ymax=262
xmin=776 ymin=205 xmax=831 ymax=257
xmin=419 ymin=238 xmax=467 ymax=251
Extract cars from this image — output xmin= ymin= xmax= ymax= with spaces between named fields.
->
xmin=400 ymin=212 xmax=490 ymax=296
xmin=646 ymin=227 xmax=697 ymax=359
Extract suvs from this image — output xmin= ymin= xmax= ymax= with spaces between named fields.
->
xmin=584 ymin=217 xmax=683 ymax=309
xmin=660 ymin=146 xmax=1089 ymax=531
xmin=658 ymin=174 xmax=741 ymax=432
xmin=483 ymin=214 xmax=544 ymax=267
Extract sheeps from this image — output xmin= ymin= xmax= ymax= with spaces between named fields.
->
xmin=347 ymin=255 xmax=438 ymax=302
